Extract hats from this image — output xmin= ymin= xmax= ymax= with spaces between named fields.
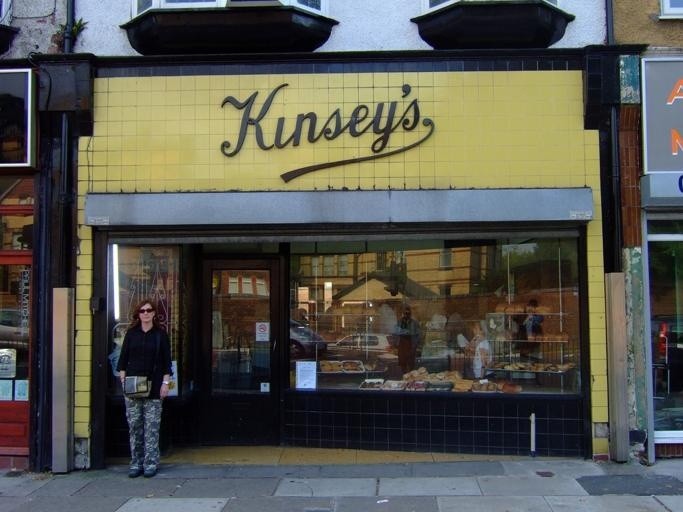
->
xmin=527 ymin=299 xmax=538 ymax=307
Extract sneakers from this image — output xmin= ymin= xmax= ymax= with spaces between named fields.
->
xmin=128 ymin=468 xmax=156 ymax=477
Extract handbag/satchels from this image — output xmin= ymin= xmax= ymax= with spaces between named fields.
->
xmin=121 ymin=375 xmax=152 ymax=399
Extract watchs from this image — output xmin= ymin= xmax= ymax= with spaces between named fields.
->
xmin=162 ymin=381 xmax=169 ymax=385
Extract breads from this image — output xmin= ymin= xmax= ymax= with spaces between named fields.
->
xmin=317 ymin=304 xmax=568 ymax=393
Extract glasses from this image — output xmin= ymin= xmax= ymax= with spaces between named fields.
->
xmin=138 ymin=308 xmax=155 ymax=313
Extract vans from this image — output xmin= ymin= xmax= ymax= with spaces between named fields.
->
xmin=291 ymin=321 xmax=326 ymax=358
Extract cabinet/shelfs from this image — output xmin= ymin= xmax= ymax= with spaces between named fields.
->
xmin=486 ymin=311 xmax=576 ymax=393
xmin=300 ymin=311 xmax=389 ymax=379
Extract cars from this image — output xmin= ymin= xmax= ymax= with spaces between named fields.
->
xmin=1 ymin=309 xmax=30 ymax=350
xmin=327 ymin=335 xmax=397 ymax=357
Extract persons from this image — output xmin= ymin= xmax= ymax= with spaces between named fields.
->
xmin=116 ymin=299 xmax=173 ymax=477
xmin=392 ymin=306 xmax=423 ymax=373
xmin=518 ymin=298 xmax=544 ymax=385
xmin=443 ymin=298 xmax=464 ymax=350
xmin=465 ymin=323 xmax=495 ymax=379
